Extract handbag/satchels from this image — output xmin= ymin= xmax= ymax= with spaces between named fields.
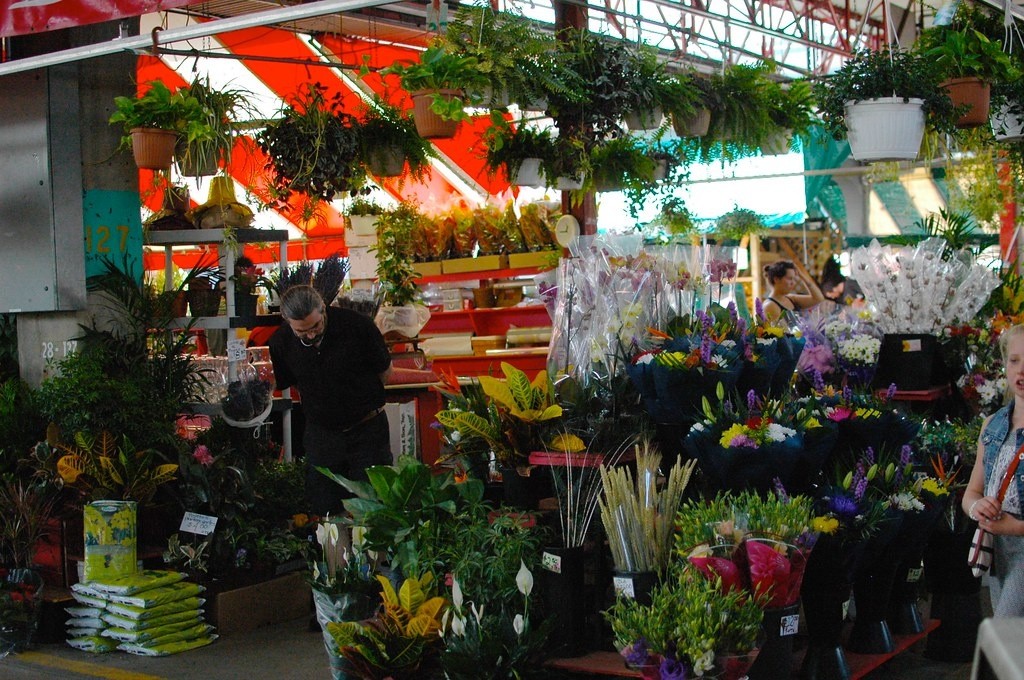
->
xmin=967 ymin=525 xmax=994 ymax=578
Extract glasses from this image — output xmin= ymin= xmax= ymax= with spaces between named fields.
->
xmin=288 ymin=315 xmax=325 ymax=337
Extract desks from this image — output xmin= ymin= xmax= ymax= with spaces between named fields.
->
xmin=551 ymin=619 xmax=942 ymax=680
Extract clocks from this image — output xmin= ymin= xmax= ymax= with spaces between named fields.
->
xmin=552 ymin=214 xmax=581 ymax=247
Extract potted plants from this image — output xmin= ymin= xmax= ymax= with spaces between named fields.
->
xmin=87 ymin=71 xmax=179 ymax=170
xmin=488 ymin=124 xmax=590 ymax=191
xmin=911 ymin=26 xmax=1023 ymax=128
xmin=171 ymin=75 xmax=261 ymax=177
xmin=593 ymin=127 xmax=659 ymax=194
xmin=811 ymin=0 xmax=972 ymax=162
xmin=596 ymin=40 xmax=670 ymax=129
xmin=645 ymin=149 xmax=679 ymax=179
xmin=376 ymin=48 xmax=495 ymax=138
xmin=355 ymin=97 xmax=439 ymax=180
xmin=671 ymin=67 xmax=748 ymax=162
xmin=757 ymin=60 xmax=822 ymax=155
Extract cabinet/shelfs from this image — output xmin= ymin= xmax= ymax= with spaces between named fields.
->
xmin=353 ymin=268 xmax=553 ymax=474
xmin=142 ymin=228 xmax=294 ymax=461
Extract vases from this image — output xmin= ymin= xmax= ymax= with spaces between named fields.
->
xmin=235 ymin=292 xmax=258 ymax=316
xmin=161 ymin=566 xmax=235 ymax=628
xmin=187 ymin=290 xmax=220 ymax=316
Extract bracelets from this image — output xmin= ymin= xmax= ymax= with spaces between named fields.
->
xmin=969 ymin=500 xmax=979 ymax=521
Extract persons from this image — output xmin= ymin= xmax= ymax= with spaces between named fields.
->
xmin=822 ymin=271 xmax=860 ymax=305
xmin=961 ymin=323 xmax=1024 ymax=620
xmin=268 ymin=285 xmax=393 ymax=633
xmin=762 ymin=261 xmax=825 ymax=322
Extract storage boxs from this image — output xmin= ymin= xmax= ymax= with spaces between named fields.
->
xmin=422 ymin=309 xmax=474 ymax=334
xmin=442 ymin=255 xmax=505 ymax=273
xmin=410 ymin=262 xmax=441 ymax=277
xmin=214 ymin=570 xmax=312 ymax=638
xmin=509 ymin=250 xmax=561 ymax=267
xmin=468 ymin=305 xmax=551 ymax=339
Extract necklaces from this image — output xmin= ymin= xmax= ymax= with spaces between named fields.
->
xmin=301 ymin=339 xmax=313 ymax=347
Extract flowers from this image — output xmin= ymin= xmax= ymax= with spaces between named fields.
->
xmin=303 ymin=201 xmax=1024 ymax=680
xmin=0 ymin=252 xmax=220 ymax=658
xmin=229 ymin=256 xmax=265 ymax=293
xmin=167 ymin=434 xmax=250 ymax=573
